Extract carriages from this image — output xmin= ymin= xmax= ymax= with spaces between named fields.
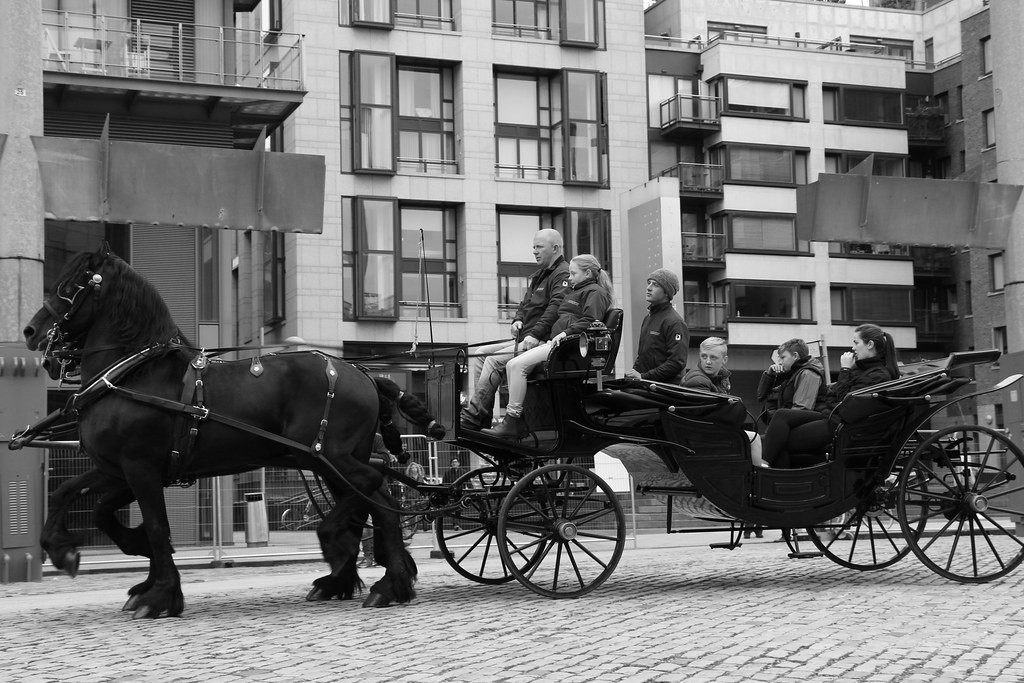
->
xmin=7 ymin=241 xmax=1024 ymax=620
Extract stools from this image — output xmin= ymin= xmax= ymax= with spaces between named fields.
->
xmin=124 ymin=34 xmax=151 ymax=78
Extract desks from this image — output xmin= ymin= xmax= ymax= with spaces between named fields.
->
xmin=73 ymin=36 xmax=113 ymax=77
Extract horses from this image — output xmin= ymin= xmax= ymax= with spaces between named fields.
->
xmin=22 ymin=238 xmax=446 ymax=619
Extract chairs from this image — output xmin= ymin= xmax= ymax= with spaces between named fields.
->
xmin=44 ymin=28 xmax=71 ymax=73
xmin=683 ymin=245 xmax=695 ymax=261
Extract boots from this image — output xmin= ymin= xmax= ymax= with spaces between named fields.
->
xmin=480 ymin=413 xmax=523 ymax=445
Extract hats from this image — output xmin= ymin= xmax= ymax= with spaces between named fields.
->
xmin=647 ymin=267 xmax=679 ymax=300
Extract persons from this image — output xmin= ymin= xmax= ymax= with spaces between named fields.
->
xmin=677 ymin=336 xmax=769 ymax=471
xmin=481 ymin=255 xmax=612 ymax=439
xmin=756 ymin=339 xmax=832 ymax=416
xmin=461 ymin=227 xmax=571 ymax=432
xmin=624 ymin=267 xmax=690 ymax=387
xmin=761 ymin=321 xmax=900 ymax=471
xmin=356 ymin=457 xmax=473 ymax=568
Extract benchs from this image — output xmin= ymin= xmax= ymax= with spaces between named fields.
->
xmin=490 ymin=307 xmax=626 ymax=392
